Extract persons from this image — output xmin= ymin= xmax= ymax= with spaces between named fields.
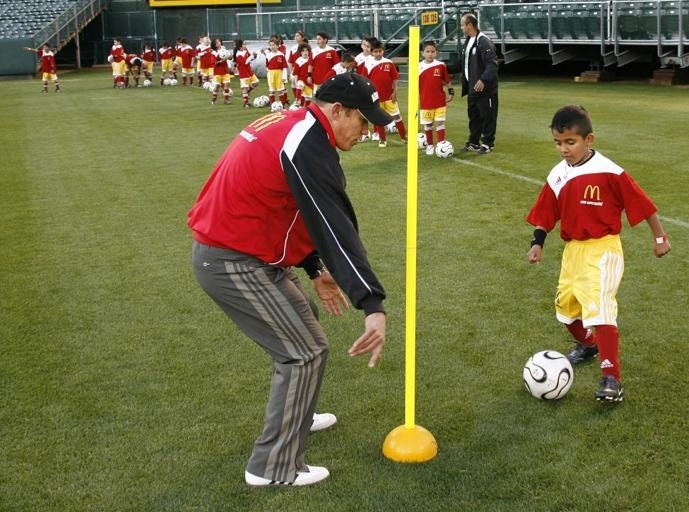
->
xmin=461 ymin=13 xmax=498 ymax=154
xmin=108 ymin=37 xmax=156 ymax=87
xmin=260 ymin=33 xmax=290 ymax=109
xmin=210 ymin=38 xmax=232 ymax=105
xmin=186 ymin=72 xmax=388 ymax=487
xmin=160 ymin=36 xmax=215 ymax=86
xmin=287 ymin=29 xmax=407 ymax=148
xmin=232 ymin=40 xmax=259 ymax=108
xmin=24 ymin=43 xmax=60 ymax=94
xmin=419 ymin=41 xmax=454 ymax=155
xmin=526 ymin=103 xmax=670 ymax=405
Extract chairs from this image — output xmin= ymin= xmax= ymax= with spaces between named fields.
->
xmin=0 ymin=0 xmax=104 ymax=63
xmin=273 ymin=0 xmax=689 ymax=40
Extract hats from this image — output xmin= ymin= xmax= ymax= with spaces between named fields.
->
xmin=315 ymin=72 xmax=394 ymax=126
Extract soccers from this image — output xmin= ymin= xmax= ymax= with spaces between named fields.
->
xmin=208 ymin=84 xmax=215 ymax=92
xmin=418 ymin=133 xmax=428 ymax=149
xmin=253 ymin=95 xmax=300 ymax=113
xmin=219 ymin=49 xmax=228 ymax=58
xmin=203 ymin=82 xmax=210 ymax=89
xmin=357 ymin=130 xmax=370 ymax=142
xmin=224 ymin=89 xmax=233 ymax=96
xmin=388 ymin=120 xmax=399 ymax=134
xmin=171 ymin=79 xmax=177 ymax=86
xmin=523 ymin=350 xmax=574 ymax=401
xmin=144 ymin=80 xmax=151 ymax=87
xmin=163 ymin=79 xmax=170 ymax=86
xmin=436 ymin=140 xmax=454 ymax=158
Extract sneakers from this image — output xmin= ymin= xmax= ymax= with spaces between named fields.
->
xmin=567 ymin=339 xmax=598 ymax=365
xmin=595 ymin=376 xmax=625 ymax=404
xmin=245 ymin=462 xmax=330 ymax=487
xmin=310 ymin=411 xmax=337 ymax=432
xmin=372 ymin=132 xmax=495 ymax=154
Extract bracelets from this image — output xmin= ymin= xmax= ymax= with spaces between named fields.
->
xmin=448 ymin=88 xmax=454 ymax=95
xmin=655 ymin=234 xmax=666 ymax=244
xmin=530 ymin=229 xmax=547 ymax=249
